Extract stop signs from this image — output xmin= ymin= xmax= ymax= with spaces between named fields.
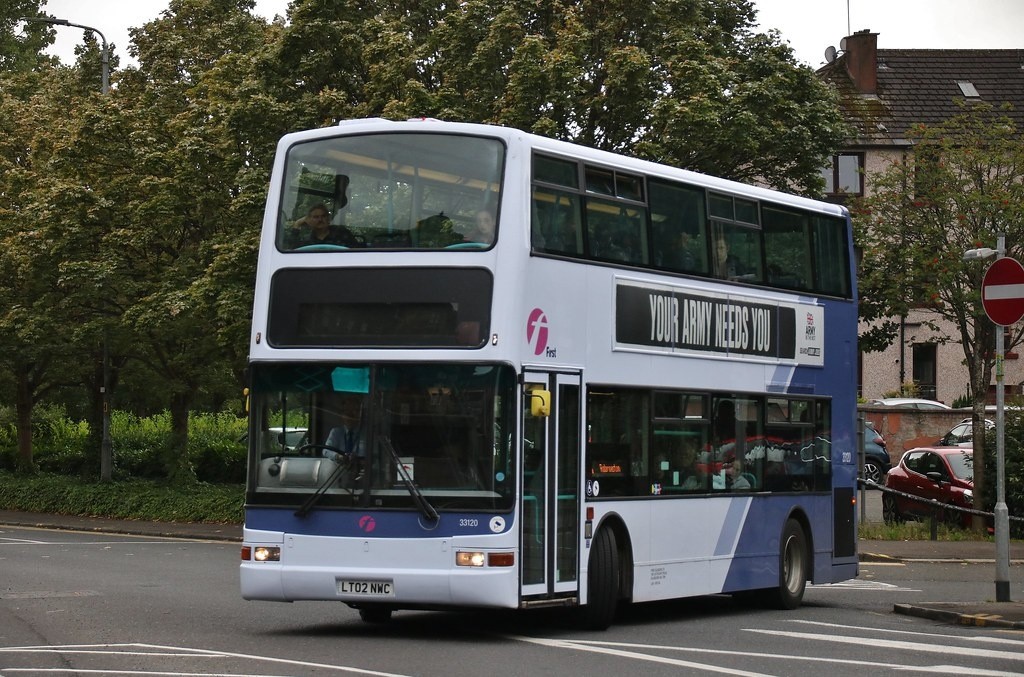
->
xmin=982 ymin=258 xmax=1024 ymax=327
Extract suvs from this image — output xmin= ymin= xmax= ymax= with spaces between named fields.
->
xmin=940 ymin=418 xmax=997 ymax=447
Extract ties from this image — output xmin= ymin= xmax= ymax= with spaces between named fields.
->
xmin=346 ymin=432 xmax=353 ymax=452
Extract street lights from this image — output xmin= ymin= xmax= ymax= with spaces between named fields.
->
xmin=962 ymin=244 xmax=1012 ymax=602
xmin=20 ymin=16 xmax=113 ymax=483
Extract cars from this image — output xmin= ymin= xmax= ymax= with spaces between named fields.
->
xmin=234 ymin=427 xmax=309 ymax=450
xmin=856 ymin=422 xmax=892 ymax=490
xmin=882 ymin=447 xmax=994 ymax=537
xmin=693 ymin=437 xmax=797 ymax=493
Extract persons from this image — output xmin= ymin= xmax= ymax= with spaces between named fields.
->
xmin=655 ymin=440 xmax=752 ymax=490
xmin=716 ymin=236 xmax=745 ymax=279
xmin=462 ymin=205 xmax=496 ymax=243
xmin=323 ymin=405 xmax=367 ymax=461
xmin=288 ymin=204 xmax=359 ymax=249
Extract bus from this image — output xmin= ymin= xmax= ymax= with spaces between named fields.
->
xmin=239 ymin=117 xmax=859 ymax=630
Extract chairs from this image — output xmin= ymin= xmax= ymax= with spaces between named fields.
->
xmin=659 ymin=472 xmax=758 ymax=493
xmin=546 ymin=238 xmax=801 ymax=289
